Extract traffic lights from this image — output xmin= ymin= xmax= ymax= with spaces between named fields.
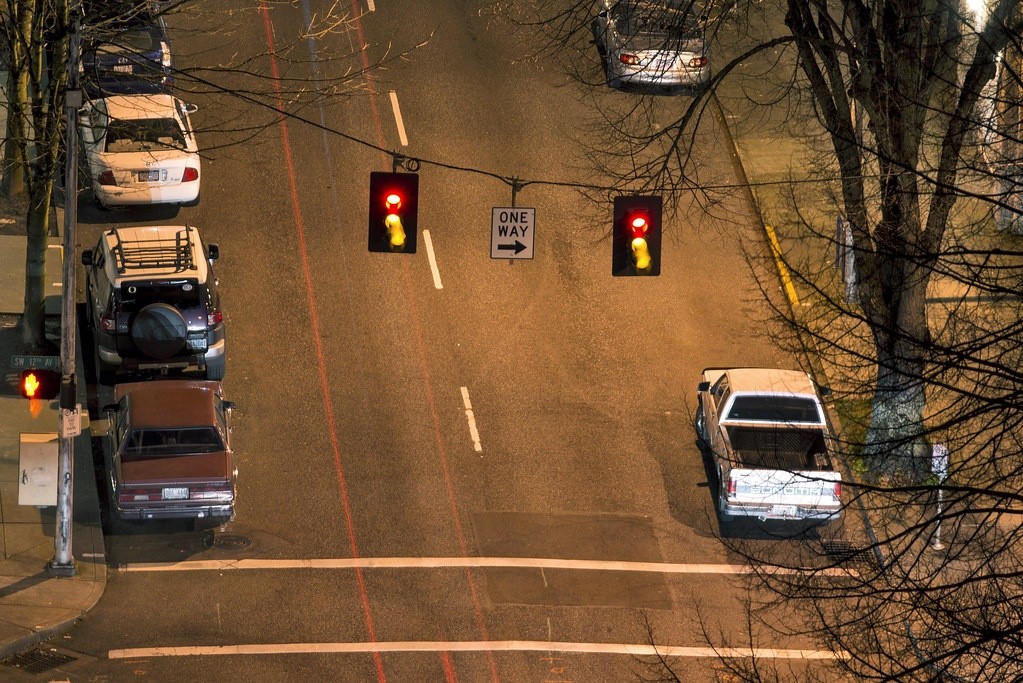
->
xmin=23 ymin=371 xmax=60 ymax=401
xmin=368 ymin=171 xmax=420 ymax=256
xmin=611 ymin=194 xmax=663 ymax=277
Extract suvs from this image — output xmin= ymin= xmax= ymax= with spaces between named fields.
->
xmin=81 ymin=223 xmax=228 ymax=379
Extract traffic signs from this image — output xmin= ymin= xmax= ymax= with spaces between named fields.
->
xmin=487 ymin=206 xmax=535 ymax=261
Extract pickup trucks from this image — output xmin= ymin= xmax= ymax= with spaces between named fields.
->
xmin=694 ymin=367 xmax=853 ymax=520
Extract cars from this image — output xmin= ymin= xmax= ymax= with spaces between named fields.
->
xmin=597 ymin=0 xmax=714 ymax=90
xmin=78 ymin=93 xmax=205 ymax=208
xmin=76 ymin=0 xmax=180 ymax=94
xmin=962 ymin=1 xmax=1013 ymax=131
xmin=108 ymin=379 xmax=239 ymax=524
